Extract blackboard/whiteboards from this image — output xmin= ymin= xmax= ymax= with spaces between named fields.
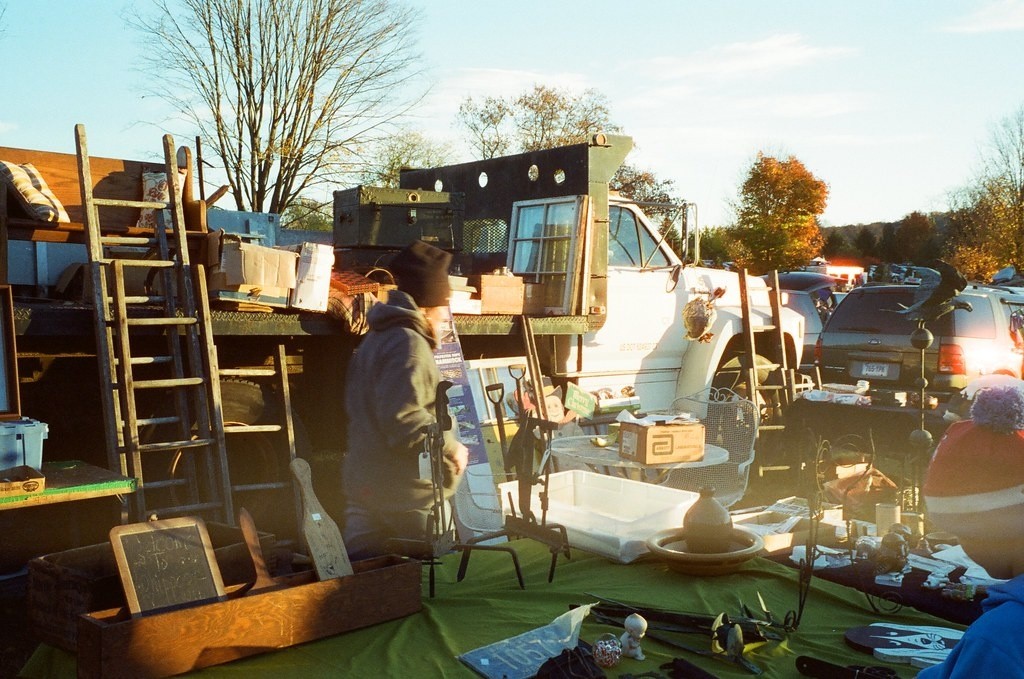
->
xmin=109 ymin=515 xmax=229 ymax=620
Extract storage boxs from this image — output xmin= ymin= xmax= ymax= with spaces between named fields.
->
xmin=618 ymin=420 xmax=706 ymax=464
xmin=76 ymin=553 xmax=423 ymax=679
xmin=204 ymin=228 xmax=298 ymax=309
xmin=0 ymin=465 xmax=45 ymax=506
xmin=497 ymin=469 xmax=701 ymax=564
xmin=594 ymin=396 xmax=641 ymax=415
xmin=330 ymin=271 xmax=379 ymax=296
xmin=448 ymin=275 xmax=468 ymax=286
xmin=449 ymin=286 xmax=477 ymax=299
xmin=272 ymin=242 xmax=334 ymax=313
xmin=448 ymin=299 xmax=481 ymax=315
xmin=0 ymin=416 xmax=49 ymax=472
xmin=377 ymin=284 xmax=399 ymax=304
xmin=463 ymin=275 xmax=524 ymax=315
xmin=23 ymin=520 xmax=277 ymax=656
xmin=333 ymin=185 xmax=466 ymax=251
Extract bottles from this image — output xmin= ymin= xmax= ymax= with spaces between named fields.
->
xmin=683 ymin=485 xmax=732 ymax=555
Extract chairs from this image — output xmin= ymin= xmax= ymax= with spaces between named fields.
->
xmin=655 ymin=387 xmax=760 ymax=511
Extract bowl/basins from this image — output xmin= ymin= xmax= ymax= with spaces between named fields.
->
xmin=645 ymin=525 xmax=764 ymax=575
xmin=925 ymin=532 xmax=957 ymax=547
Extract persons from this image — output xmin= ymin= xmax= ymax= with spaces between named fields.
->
xmin=619 ymin=613 xmax=647 ymax=660
xmin=913 ymin=386 xmax=1024 ymax=679
xmin=342 ymin=240 xmax=468 ymax=561
xmin=810 ymin=287 xmax=837 ymax=324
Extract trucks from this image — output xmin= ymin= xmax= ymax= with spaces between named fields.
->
xmin=0 ymin=130 xmax=809 ymax=581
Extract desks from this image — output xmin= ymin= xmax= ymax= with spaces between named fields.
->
xmin=0 ymin=459 xmax=141 ymax=582
xmin=19 ymin=536 xmax=973 ymax=679
xmin=546 ymin=435 xmax=729 ymax=485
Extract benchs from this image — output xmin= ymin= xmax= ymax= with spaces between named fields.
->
xmin=0 ymin=145 xmax=209 ymax=306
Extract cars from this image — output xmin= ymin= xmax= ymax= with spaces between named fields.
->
xmin=755 ymin=262 xmax=1024 ymax=502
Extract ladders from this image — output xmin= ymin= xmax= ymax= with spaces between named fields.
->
xmin=738 ymin=267 xmax=822 ymax=477
xmin=208 ymin=344 xmax=304 ymax=529
xmin=74 ymin=124 xmax=235 ymax=530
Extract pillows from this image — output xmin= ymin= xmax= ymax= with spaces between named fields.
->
xmin=136 ymin=169 xmax=188 ymax=228
xmin=0 ymin=160 xmax=71 ymax=223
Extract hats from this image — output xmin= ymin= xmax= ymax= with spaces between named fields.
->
xmin=388 ymin=240 xmax=452 ymax=307
xmin=923 ymin=385 xmax=1024 ymax=539
